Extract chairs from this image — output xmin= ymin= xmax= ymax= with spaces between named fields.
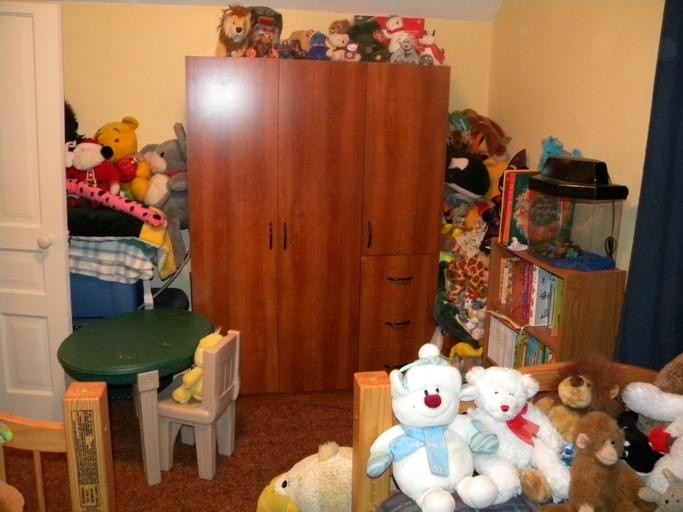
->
xmin=158 ymin=329 xmax=241 ymax=480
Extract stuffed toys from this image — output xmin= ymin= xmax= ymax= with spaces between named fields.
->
xmin=382 ymin=16 xmax=408 ymax=52
xmin=291 ymin=30 xmax=312 ymax=53
xmin=544 ymin=413 xmax=652 ymax=511
xmin=467 ymin=365 xmax=572 ymax=503
xmin=326 ymin=32 xmax=361 ymax=62
xmin=636 ymin=470 xmax=683 ymax=511
xmin=95 ymin=117 xmax=151 ymax=201
xmin=65 ymin=138 xmax=120 ymax=196
xmin=134 ymin=122 xmax=188 ymax=268
xmin=309 ymin=32 xmax=328 ymax=60
xmin=390 ymin=40 xmax=418 ymax=64
xmin=257 ymin=442 xmax=352 ymax=512
xmin=520 ymin=351 xmax=624 ymax=503
xmin=173 ymin=327 xmax=226 ymax=403
xmin=420 ymin=29 xmax=439 ymax=65
xmin=366 ymin=344 xmax=497 ymax=512
xmin=621 ymin=383 xmax=683 ymax=494
xmin=349 ymin=21 xmax=388 ymax=62
xmin=245 ymin=7 xmax=281 ymax=59
xmin=216 ymin=4 xmax=250 ymax=58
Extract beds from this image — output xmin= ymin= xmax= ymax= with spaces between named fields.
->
xmin=352 ymin=353 xmax=660 ymax=512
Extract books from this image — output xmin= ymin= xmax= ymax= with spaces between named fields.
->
xmin=487 ymin=317 xmax=552 ymax=368
xmin=499 ymin=257 xmax=564 ymax=337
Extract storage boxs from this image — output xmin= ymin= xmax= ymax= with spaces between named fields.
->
xmin=69 ymin=236 xmax=138 ymax=317
xmin=528 ymin=155 xmax=629 ymax=270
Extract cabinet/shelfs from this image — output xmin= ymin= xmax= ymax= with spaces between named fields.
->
xmin=185 ymin=55 xmax=366 ymax=394
xmin=481 ymin=237 xmax=627 ymax=369
xmin=362 ymin=60 xmax=452 ymax=255
xmin=358 ymin=253 xmax=439 ymax=375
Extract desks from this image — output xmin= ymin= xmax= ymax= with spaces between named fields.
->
xmin=57 ymin=310 xmax=213 ymax=486
